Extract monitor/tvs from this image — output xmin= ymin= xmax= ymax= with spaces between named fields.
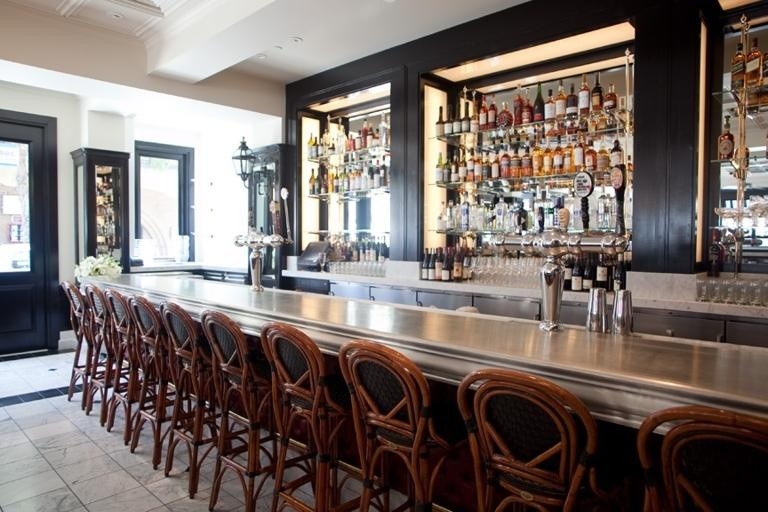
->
xmin=297 ymin=242 xmax=330 ymax=268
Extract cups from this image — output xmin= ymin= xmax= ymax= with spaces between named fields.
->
xmin=695 ymin=271 xmax=766 ymax=308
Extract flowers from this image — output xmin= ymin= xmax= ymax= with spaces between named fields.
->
xmin=73 ymin=253 xmax=123 ymax=277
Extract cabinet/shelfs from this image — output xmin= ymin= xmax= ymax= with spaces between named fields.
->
xmin=285 ymin=61 xmax=407 ymax=261
xmin=70 ymin=148 xmax=130 ymax=273
xmin=664 ymin=0 xmax=768 ymax=273
xmin=411 ymin=17 xmax=664 ymax=262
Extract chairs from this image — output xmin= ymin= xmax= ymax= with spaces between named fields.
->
xmin=459 ymin=368 xmax=603 ymax=510
xmin=85 ymin=288 xmax=119 ymax=428
xmin=109 ymin=295 xmax=146 ymax=444
xmin=339 ymin=343 xmax=477 ymax=510
xmin=67 ymin=286 xmax=98 ymax=410
xmin=163 ymin=306 xmax=241 ymax=500
xmin=202 ymin=313 xmax=315 ymax=511
xmin=261 ymin=327 xmax=397 ymax=512
xmin=130 ymin=301 xmax=197 ymax=470
xmin=637 ymin=405 xmax=768 ymax=510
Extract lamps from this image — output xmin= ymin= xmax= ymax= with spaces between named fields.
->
xmin=230 ymin=136 xmax=269 ymax=193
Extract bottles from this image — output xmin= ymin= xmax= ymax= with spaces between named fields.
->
xmin=587 ymin=285 xmax=608 ymax=334
xmin=729 ymin=43 xmax=747 ymax=89
xmin=706 ymin=228 xmax=722 ymax=276
xmin=308 ymin=111 xmax=391 ymax=196
xmin=744 ymin=37 xmax=764 ymax=87
xmin=611 ymin=287 xmax=632 ymax=335
xmin=729 ymin=145 xmax=750 ymax=183
xmin=715 ymin=114 xmax=745 ymax=161
xmin=748 ymin=90 xmax=763 ymax=115
xmin=312 ymin=229 xmax=391 ymax=276
xmin=421 ymin=70 xmax=631 ymax=291
